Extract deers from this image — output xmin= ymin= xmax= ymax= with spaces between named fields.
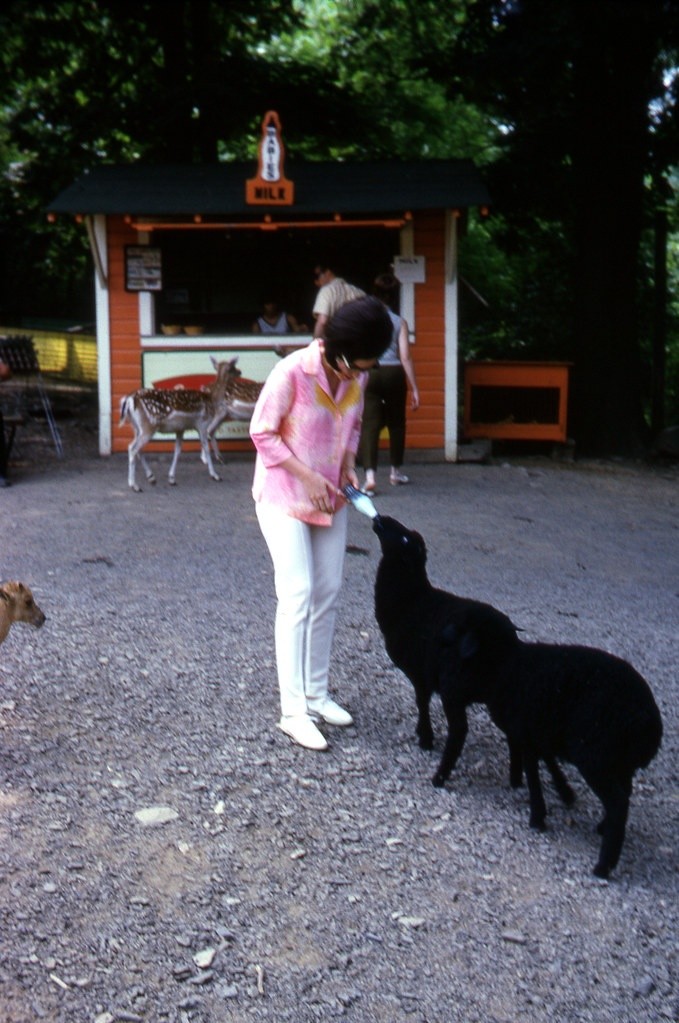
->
xmin=119 ymin=354 xmax=265 ymax=493
xmin=0 ymin=580 xmax=46 ymax=642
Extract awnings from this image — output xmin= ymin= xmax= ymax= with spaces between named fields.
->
xmin=37 ymin=158 xmax=494 ymax=215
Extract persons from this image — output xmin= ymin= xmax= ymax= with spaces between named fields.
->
xmin=360 ymin=272 xmax=420 ymax=496
xmin=312 ymin=257 xmax=367 ymax=338
xmin=249 ymin=293 xmax=395 ymax=750
xmin=251 ymin=295 xmax=310 ymax=333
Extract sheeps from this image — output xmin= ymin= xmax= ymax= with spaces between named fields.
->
xmin=371 ymin=514 xmax=663 ymax=875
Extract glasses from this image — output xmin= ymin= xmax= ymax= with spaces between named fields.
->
xmin=313 ymin=272 xmax=322 ymax=280
xmin=340 ymin=354 xmax=380 ymax=373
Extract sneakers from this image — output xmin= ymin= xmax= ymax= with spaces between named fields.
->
xmin=280 ymin=715 xmax=327 ymax=750
xmin=308 ymin=700 xmax=353 ymax=726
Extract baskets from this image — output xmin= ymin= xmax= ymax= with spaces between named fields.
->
xmin=161 ymin=325 xmax=180 ymax=336
xmin=182 ymin=326 xmax=202 ymax=336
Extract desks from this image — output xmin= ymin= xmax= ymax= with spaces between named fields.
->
xmin=0 ymin=374 xmax=92 ymax=461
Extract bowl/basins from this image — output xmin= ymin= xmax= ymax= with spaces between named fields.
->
xmin=160 ymin=324 xmax=182 ymax=334
xmin=183 ymin=325 xmax=206 ymax=336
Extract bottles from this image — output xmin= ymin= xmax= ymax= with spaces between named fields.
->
xmin=261 ymin=128 xmax=281 ymax=182
xmin=346 ymin=486 xmax=383 ymax=524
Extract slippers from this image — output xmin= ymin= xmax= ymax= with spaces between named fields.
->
xmin=390 ymin=475 xmax=409 ymax=486
xmin=359 ymin=486 xmax=375 ymax=497
